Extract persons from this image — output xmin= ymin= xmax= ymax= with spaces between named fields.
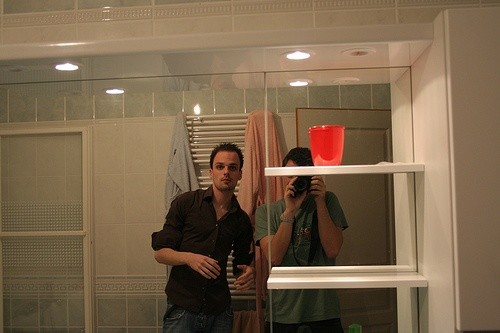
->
xmin=151 ymin=142 xmax=254 ymax=332
xmin=255 ymin=146 xmax=349 ymax=332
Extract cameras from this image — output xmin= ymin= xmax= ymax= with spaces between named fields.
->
xmin=290 ymin=176 xmax=313 ymax=199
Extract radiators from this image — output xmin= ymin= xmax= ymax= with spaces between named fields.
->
xmin=187 ymin=114 xmax=267 ymax=300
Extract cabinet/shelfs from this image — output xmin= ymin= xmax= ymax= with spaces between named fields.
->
xmin=264 ymin=164 xmax=430 ymax=289
xmin=411 ymin=6 xmax=500 ymax=333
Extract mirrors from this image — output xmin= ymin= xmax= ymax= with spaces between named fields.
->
xmin=0 ymin=66 xmax=419 ymax=333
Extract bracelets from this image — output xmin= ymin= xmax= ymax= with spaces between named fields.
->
xmin=280 ymin=212 xmax=296 ymax=223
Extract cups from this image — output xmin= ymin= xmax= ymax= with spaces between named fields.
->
xmin=308 ymin=125 xmax=346 ymax=166
